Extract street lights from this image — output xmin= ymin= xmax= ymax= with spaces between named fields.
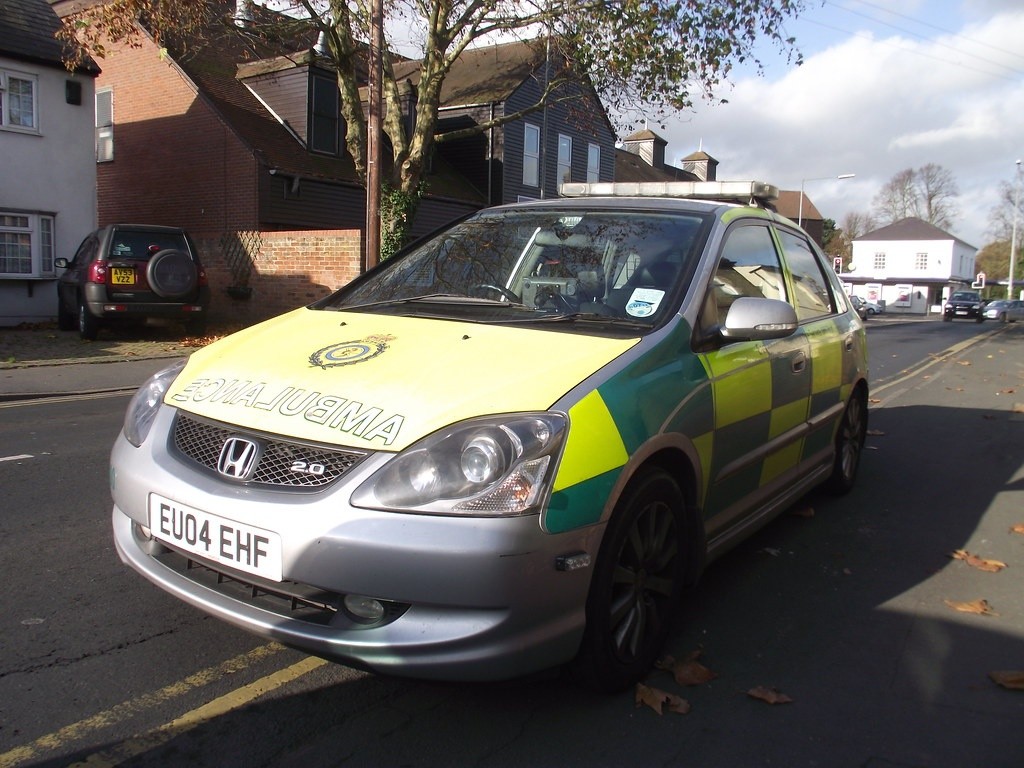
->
xmin=798 ymin=173 xmax=856 ymax=229
xmin=1008 ymin=159 xmax=1021 ymax=298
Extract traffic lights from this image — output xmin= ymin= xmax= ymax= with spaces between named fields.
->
xmin=834 ymin=257 xmax=841 ymax=275
xmin=972 ymin=273 xmax=986 ymax=288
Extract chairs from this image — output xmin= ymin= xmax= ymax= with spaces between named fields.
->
xmin=536 ymin=262 xmax=677 ymax=313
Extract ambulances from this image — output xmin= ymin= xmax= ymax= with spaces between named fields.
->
xmin=106 ymin=181 xmax=871 ymax=685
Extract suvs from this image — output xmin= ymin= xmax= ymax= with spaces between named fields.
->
xmin=54 ymin=224 xmax=210 ymax=341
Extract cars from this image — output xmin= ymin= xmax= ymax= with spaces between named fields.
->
xmin=848 ymin=295 xmax=868 ymax=321
xmin=983 ymin=299 xmax=1024 ymax=322
xmin=942 ymin=292 xmax=984 ymax=323
xmin=860 ymin=297 xmax=883 ymax=314
